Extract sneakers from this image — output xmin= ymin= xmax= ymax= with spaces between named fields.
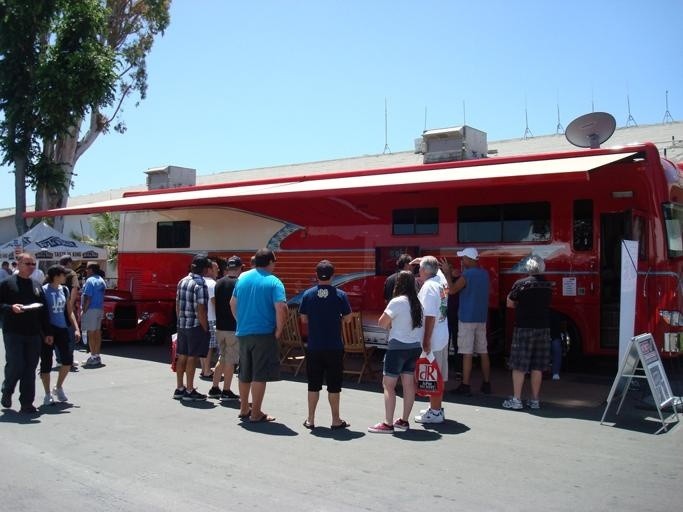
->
xmin=392 ymin=418 xmax=410 ymax=430
xmin=366 ymin=421 xmax=394 ymax=434
xmin=181 ymin=387 xmax=208 ymax=402
xmin=0 ymin=391 xmax=12 ymax=409
xmin=55 ymin=361 xmax=79 ymax=367
xmin=42 ymin=394 xmax=54 ymax=407
xmin=479 ymin=382 xmax=491 ymax=394
xmin=418 ymin=406 xmax=444 ymax=418
xmin=413 ymin=412 xmax=444 ymax=424
xmin=524 ymin=398 xmax=540 ymax=409
xmin=19 ymin=403 xmax=35 ymax=412
xmin=79 ymin=356 xmax=102 ymax=367
xmin=447 ymin=381 xmax=471 ymax=395
xmin=172 ymin=386 xmax=186 ymax=399
xmin=51 ymin=385 xmax=69 ymax=403
xmin=500 ymin=397 xmax=524 ymax=409
xmin=218 ymin=390 xmax=240 ymax=402
xmin=206 ymin=386 xmax=222 ymax=398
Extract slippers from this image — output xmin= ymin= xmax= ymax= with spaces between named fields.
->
xmin=248 ymin=412 xmax=276 ymax=423
xmin=200 ymin=369 xmax=225 ymax=383
xmin=236 ymin=408 xmax=251 ymax=419
xmin=329 ymin=419 xmax=351 ymax=429
xmin=302 ymin=417 xmax=314 ymax=429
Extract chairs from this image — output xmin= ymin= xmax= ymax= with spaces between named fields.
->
xmin=340 ymin=312 xmax=377 ymax=382
xmin=278 ymin=305 xmax=306 ymax=378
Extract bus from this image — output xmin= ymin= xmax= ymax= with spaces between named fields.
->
xmin=19 ymin=140 xmax=682 ymax=379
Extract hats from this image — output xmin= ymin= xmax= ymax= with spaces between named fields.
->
xmin=313 ymin=260 xmax=335 ymax=280
xmin=46 ymin=264 xmax=71 ymax=275
xmin=456 ymin=247 xmax=479 ymax=261
xmin=225 ymin=255 xmax=241 ymax=270
xmin=190 ymin=253 xmax=212 ymax=268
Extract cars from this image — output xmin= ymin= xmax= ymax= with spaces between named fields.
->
xmin=67 ymin=275 xmax=154 ymax=350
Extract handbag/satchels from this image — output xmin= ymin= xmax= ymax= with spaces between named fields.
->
xmin=170 ymin=332 xmax=177 ymax=372
xmin=61 ymin=285 xmax=72 ymax=327
xmin=414 ymin=350 xmax=444 ymax=399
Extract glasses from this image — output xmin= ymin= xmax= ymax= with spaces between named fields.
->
xmin=19 ymin=261 xmax=35 ymax=265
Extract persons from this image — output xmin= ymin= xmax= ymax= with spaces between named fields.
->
xmin=206 ymin=255 xmax=243 ymax=402
xmin=229 ymin=247 xmax=290 ymax=422
xmin=298 ymin=260 xmax=353 ymax=430
xmin=366 ymin=246 xmax=492 ymax=434
xmin=1 ymin=252 xmax=108 ymax=415
xmin=173 ymin=253 xmax=211 ymax=401
xmin=199 ymin=260 xmax=224 ymax=382
xmin=502 ymin=255 xmax=554 ymax=410
